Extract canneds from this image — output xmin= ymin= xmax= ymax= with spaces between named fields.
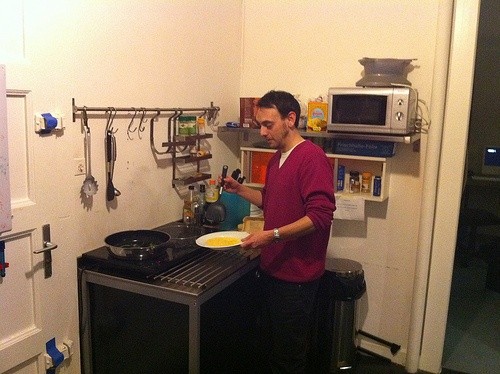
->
xmin=349 ymin=170 xmax=371 ymax=193
xmin=178 ymin=115 xmax=196 ymax=136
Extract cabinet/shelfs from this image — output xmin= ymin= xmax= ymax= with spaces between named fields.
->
xmin=240 ymin=147 xmax=390 ymax=203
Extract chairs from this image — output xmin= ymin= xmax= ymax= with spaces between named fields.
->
xmin=459 ymin=184 xmax=500 ymax=251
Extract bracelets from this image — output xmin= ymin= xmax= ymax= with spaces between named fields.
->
xmin=273 ymin=228 xmax=280 ymax=241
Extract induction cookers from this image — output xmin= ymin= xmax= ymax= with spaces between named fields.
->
xmin=81 ymin=221 xmax=220 ymax=273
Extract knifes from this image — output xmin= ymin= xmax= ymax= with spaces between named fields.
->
xmin=231 ymin=168 xmax=246 ymax=184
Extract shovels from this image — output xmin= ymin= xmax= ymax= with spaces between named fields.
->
xmin=206 ymin=165 xmax=229 ymax=223
xmin=106 ymin=130 xmax=115 ymax=201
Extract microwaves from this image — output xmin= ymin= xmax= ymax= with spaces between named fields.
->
xmin=328 ymin=87 xmax=418 ymax=135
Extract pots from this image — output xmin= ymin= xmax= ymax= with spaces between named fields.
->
xmin=104 ymin=230 xmax=178 ymax=261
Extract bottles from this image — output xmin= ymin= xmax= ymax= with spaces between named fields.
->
xmin=183 ymin=185 xmax=197 ymax=224
xmin=198 ymin=118 xmax=206 ymax=135
xmin=350 ymin=170 xmax=359 ymax=193
xmin=337 ymin=164 xmax=345 ymax=191
xmin=198 ymin=179 xmax=218 ymax=216
xmin=361 ymin=173 xmax=372 ymax=193
xmin=373 ymin=176 xmax=381 ymax=196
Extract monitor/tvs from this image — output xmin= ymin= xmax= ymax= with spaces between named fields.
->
xmin=482 ymin=146 xmax=500 ymax=177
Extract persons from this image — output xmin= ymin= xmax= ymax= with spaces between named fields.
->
xmin=218 ymin=91 xmax=336 ymax=374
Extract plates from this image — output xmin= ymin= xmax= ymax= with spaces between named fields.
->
xmin=195 ymin=231 xmax=253 ymax=251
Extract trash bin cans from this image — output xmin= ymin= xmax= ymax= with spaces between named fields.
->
xmin=313 ymin=257 xmax=366 ymax=373
